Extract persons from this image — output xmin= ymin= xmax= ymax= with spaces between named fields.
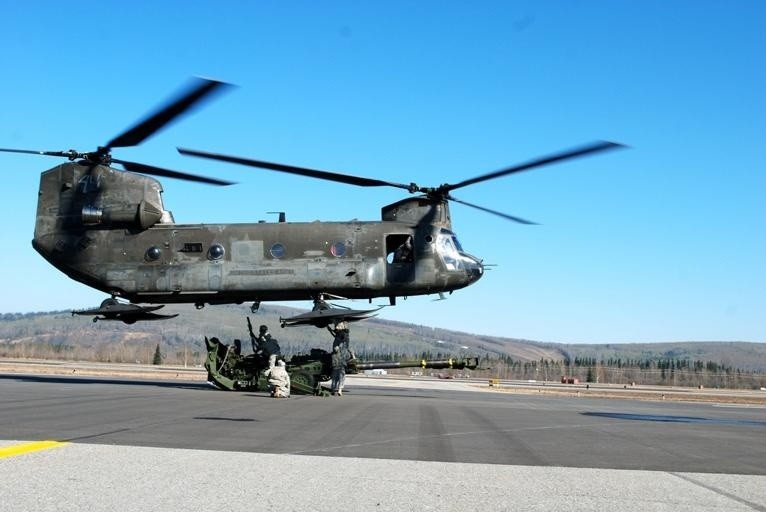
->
xmin=331 ymin=346 xmax=352 ymax=396
xmin=333 ymin=317 xmax=350 ymax=348
xmin=249 ymin=326 xmax=280 ymax=358
xmin=264 ymin=361 xmax=292 ymax=399
xmin=392 ymin=235 xmax=412 ymax=263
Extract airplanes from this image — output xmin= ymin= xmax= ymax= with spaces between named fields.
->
xmin=0 ymin=76 xmax=632 ymax=327
